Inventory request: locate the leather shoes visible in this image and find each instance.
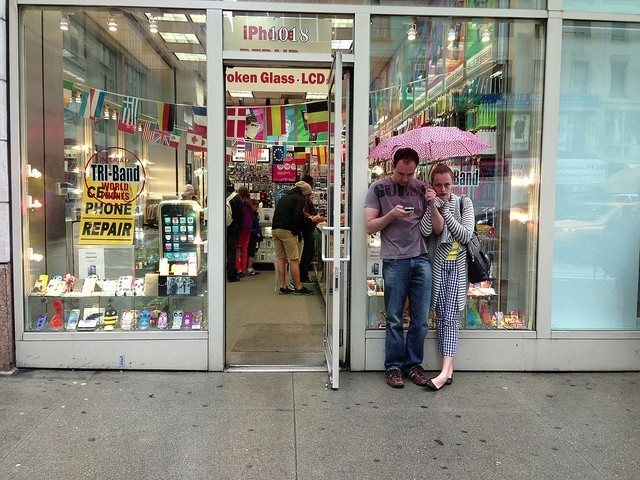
[426,375,444,390]
[445,373,453,385]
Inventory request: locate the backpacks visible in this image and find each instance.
[225,191,238,226]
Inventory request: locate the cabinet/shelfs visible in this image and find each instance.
[312,222,326,306]
[368,285,499,330]
[29,292,206,330]
[254,224,319,270]
[75,229,159,279]
[26,176,43,263]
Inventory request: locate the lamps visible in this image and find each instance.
[148,16,158,33]
[479,23,491,42]
[407,23,417,41]
[102,105,109,120]
[111,110,117,120]
[107,12,118,32]
[66,92,74,102]
[138,122,142,132]
[58,11,69,30]
[447,24,456,41]
[76,90,82,103]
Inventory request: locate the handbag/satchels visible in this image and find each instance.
[466,233,491,284]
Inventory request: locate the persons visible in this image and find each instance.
[299,175,323,285]
[235,187,256,277]
[271,181,324,296]
[226,179,244,282]
[419,163,475,391]
[364,148,433,387]
[246,199,261,276]
[182,184,201,207]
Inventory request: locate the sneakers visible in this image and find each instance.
[288,283,291,289]
[229,276,240,282]
[240,272,245,277]
[291,285,295,290]
[246,268,257,276]
[279,288,294,295]
[293,286,315,295]
[387,370,405,387]
[301,279,317,285]
[405,366,428,385]
[253,268,260,274]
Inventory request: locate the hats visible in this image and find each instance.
[296,180,312,195]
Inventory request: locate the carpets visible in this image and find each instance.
[231,322,326,352]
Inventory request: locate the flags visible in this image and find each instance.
[294,147,306,165]
[118,112,135,134]
[330,102,334,133]
[63,80,73,108]
[186,129,203,152]
[225,138,232,163]
[330,147,334,164]
[153,123,171,147]
[316,146,328,164]
[79,91,96,120]
[272,146,284,164]
[226,108,246,137]
[192,106,207,139]
[265,106,286,136]
[157,102,175,131]
[306,102,328,133]
[83,88,105,119]
[142,121,156,140]
[169,129,182,148]
[201,138,207,152]
[245,142,258,164]
[121,96,140,127]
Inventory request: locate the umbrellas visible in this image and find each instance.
[365,127,493,189]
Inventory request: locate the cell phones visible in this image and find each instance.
[157,199,201,273]
[92,265,96,275]
[87,265,92,276]
[68,309,80,327]
[405,206,415,211]
[374,262,379,275]
